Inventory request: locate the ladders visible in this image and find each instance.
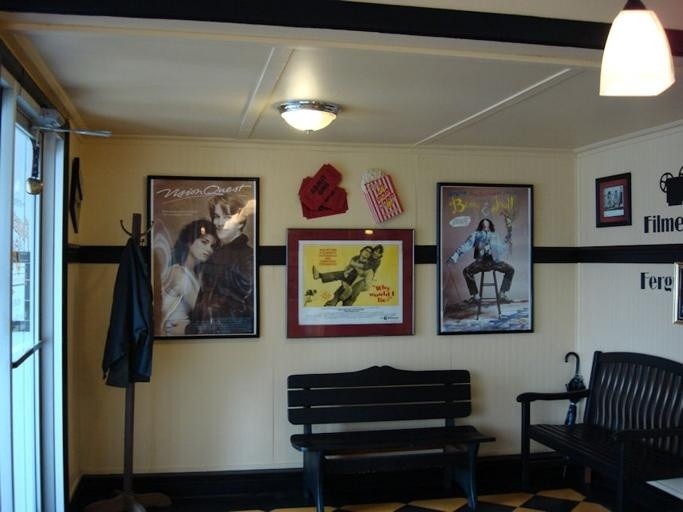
[477,270,501,320]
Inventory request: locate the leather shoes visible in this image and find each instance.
[463,297,480,305]
[497,294,512,303]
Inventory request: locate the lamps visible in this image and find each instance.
[598,0,677,99]
[277,100,339,136]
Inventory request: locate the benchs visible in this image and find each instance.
[516,351,683,512]
[287,365,497,511]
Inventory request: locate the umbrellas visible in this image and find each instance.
[558,352,585,477]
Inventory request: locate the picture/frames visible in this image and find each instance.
[436,182,534,334]
[287,228,415,338]
[595,173,631,227]
[147,175,259,338]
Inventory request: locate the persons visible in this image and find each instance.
[322,246,374,307]
[445,219,519,307]
[310,243,385,300]
[159,218,218,335]
[163,196,253,334]
